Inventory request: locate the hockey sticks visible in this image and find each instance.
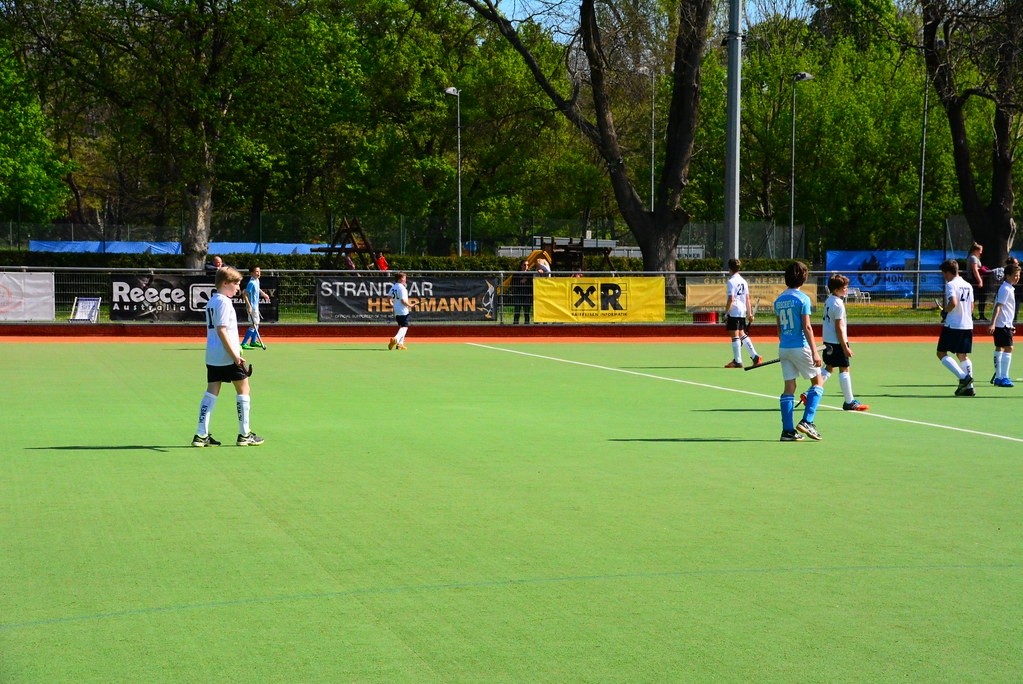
[741,296,761,346]
[241,361,253,377]
[744,344,834,372]
[934,298,943,310]
[250,313,267,350]
[990,327,1016,383]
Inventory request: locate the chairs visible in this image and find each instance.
[825,286,871,304]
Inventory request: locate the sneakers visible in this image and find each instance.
[843,400,869,411]
[250,342,262,347]
[724,359,742,368]
[800,393,807,406]
[388,337,396,350]
[780,429,804,441]
[999,378,1013,387]
[752,355,762,367]
[236,432,265,446]
[396,344,407,349]
[796,419,822,440]
[240,344,254,349]
[994,378,1001,385]
[964,388,976,396]
[192,434,222,446]
[954,375,974,395]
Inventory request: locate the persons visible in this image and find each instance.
[967,242,990,322]
[368,252,391,270]
[1005,256,1018,322]
[987,265,1021,387]
[937,259,975,396]
[241,265,270,350]
[388,272,415,350]
[774,260,825,440]
[191,266,264,447]
[205,256,227,275]
[510,257,584,325]
[346,253,355,269]
[723,259,763,368]
[800,272,869,412]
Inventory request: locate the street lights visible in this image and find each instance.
[789,72,813,258]
[445,87,461,259]
